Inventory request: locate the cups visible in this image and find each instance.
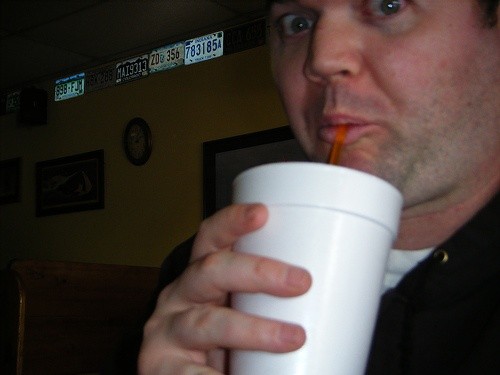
[229,161,404,375]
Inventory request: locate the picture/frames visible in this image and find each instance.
[203,123,312,219]
[0,157,22,205]
[35,149,105,216]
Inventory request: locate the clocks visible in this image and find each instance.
[124,117,153,166]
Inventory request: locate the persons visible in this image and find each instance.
[141,0,499,374]
